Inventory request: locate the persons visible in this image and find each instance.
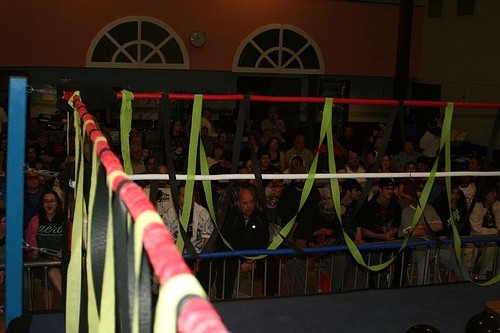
[0,207,7,285]
[0,105,500,224]
[25,190,72,310]
[288,184,348,294]
[356,178,411,287]
[334,178,389,289]
[439,186,473,282]
[162,184,217,295]
[401,187,469,285]
[469,182,500,282]
[215,188,279,299]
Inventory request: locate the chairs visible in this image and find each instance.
[182,238,491,287]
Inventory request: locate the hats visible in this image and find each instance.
[379,179,394,188]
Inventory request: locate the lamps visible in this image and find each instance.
[189,29,206,47]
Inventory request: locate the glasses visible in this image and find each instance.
[43,199,57,205]
